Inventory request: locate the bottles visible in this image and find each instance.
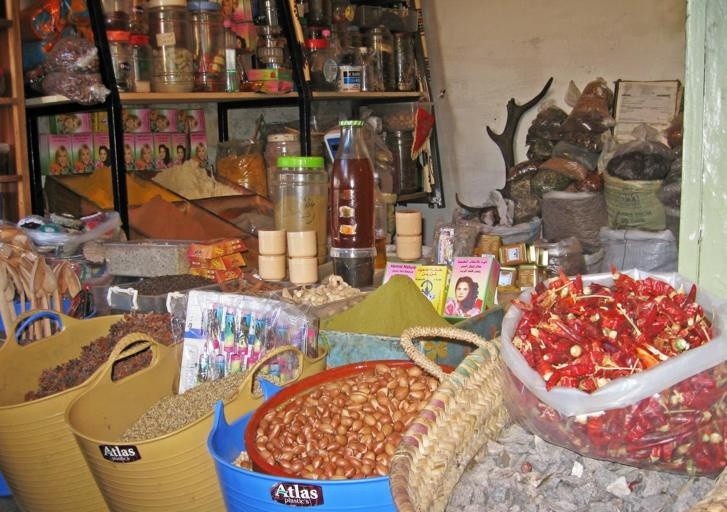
[387,129,419,195]
[304,0,417,92]
[105,1,226,93]
[330,120,375,249]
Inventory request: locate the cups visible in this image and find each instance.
[258,227,318,286]
[395,236,422,261]
[395,209,422,236]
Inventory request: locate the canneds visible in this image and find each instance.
[147,0,239,92]
[335,64,362,92]
[478,235,549,289]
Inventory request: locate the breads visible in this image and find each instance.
[0,223,81,301]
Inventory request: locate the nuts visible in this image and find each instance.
[240,363,441,480]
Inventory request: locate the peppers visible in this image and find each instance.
[507,263,727,477]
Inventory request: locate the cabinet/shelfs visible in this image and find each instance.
[1,0,445,244]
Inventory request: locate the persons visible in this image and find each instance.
[444,277,481,318]
[50,111,206,176]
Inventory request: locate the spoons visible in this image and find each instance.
[0,257,83,342]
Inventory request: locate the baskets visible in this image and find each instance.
[390,326,727,512]
